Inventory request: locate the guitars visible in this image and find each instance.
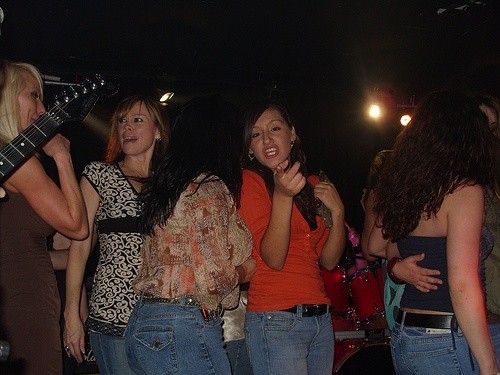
[0,74,120,199]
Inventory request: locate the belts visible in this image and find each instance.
[142,293,198,306]
[277,303,333,319]
[396,308,460,333]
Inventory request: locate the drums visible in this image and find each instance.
[332,338,396,375]
[319,265,388,328]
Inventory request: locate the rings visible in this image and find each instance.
[64,345,70,353]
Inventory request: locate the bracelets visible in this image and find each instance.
[386,257,406,285]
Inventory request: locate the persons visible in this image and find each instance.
[232,102,345,375]
[62,95,171,375]
[48,232,99,324]
[124,102,258,375]
[367,93,500,375]
[344,147,407,336]
[385,96,500,371]
[0,62,89,375]
